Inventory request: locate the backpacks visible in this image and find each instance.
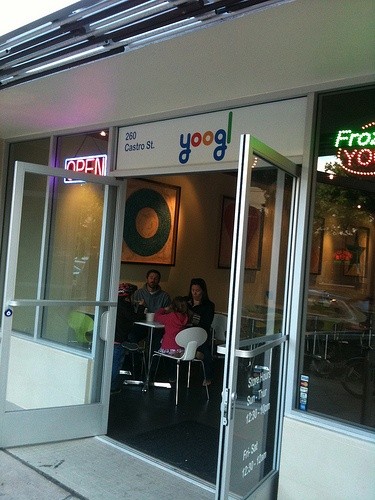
[118,283,138,302]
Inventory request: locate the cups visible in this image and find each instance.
[147,313,155,321]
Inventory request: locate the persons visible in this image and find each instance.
[104,270,217,395]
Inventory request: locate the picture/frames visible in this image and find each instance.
[343,225,370,278]
[218,195,264,271]
[310,218,325,275]
[116,177,181,266]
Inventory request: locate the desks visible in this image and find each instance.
[76,304,375,392]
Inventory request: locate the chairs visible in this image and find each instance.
[141,327,209,406]
[66,311,94,350]
[210,314,227,359]
